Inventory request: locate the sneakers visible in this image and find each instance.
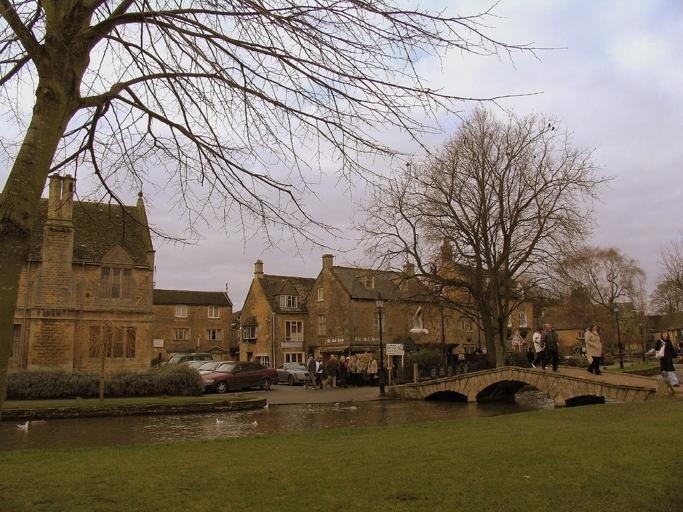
[531,362,536,368]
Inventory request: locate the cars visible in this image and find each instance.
[645,348,656,355]
[198,360,280,394]
[272,361,310,386]
[167,352,226,378]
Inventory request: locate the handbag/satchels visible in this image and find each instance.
[656,345,665,360]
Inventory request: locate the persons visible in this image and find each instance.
[585,323,603,374]
[530,327,549,369]
[303,351,398,390]
[541,321,562,373]
[593,321,601,375]
[655,330,681,387]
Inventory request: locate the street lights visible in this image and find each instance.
[372,291,388,396]
[433,282,448,370]
[613,302,626,369]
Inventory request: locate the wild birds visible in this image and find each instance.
[215,417,224,424]
[252,420,258,426]
[263,403,269,409]
[15,420,31,432]
